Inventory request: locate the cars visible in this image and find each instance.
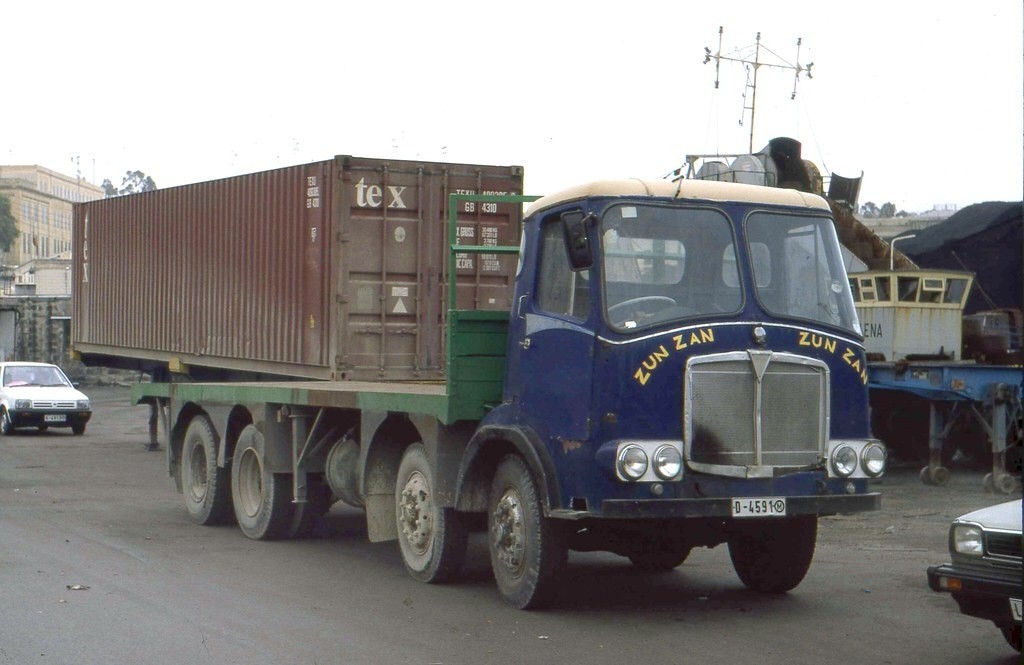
[927,497,1024,651]
[0,362,93,434]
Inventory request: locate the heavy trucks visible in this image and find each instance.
[67,154,886,613]
